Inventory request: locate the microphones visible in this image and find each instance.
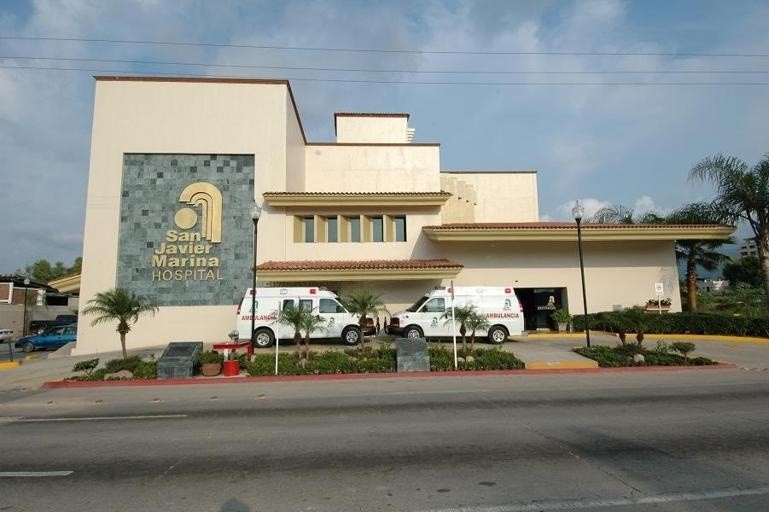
[223,360,240,376]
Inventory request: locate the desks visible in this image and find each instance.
[246,199,262,355]
[570,200,591,347]
[23,278,30,337]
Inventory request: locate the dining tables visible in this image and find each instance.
[197,350,222,376]
[551,309,572,331]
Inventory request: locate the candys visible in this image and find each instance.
[383,285,524,345]
[233,286,381,346]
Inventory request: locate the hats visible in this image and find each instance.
[15,325,79,354]
[0,327,14,344]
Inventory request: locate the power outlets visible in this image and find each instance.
[30,315,78,335]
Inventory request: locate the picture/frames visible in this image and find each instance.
[212,340,253,361]
